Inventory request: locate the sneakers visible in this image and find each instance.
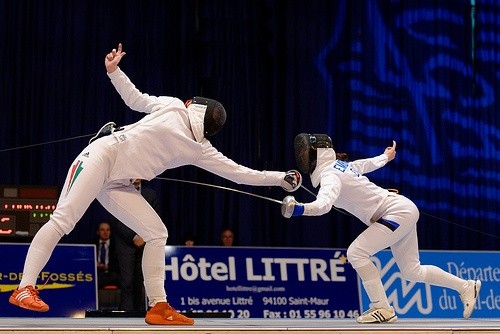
[144,302,195,325]
[458,280,481,319]
[8,285,50,313]
[356,307,398,324]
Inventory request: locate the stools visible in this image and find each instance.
[99,284,121,311]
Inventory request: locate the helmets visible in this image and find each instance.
[295,133,333,173]
[192,96,226,138]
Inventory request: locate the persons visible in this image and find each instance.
[93,180,160,311]
[183,235,197,245]
[221,229,234,246]
[292,134,481,323]
[9,44,297,325]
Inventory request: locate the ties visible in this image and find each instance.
[100,243,106,268]
